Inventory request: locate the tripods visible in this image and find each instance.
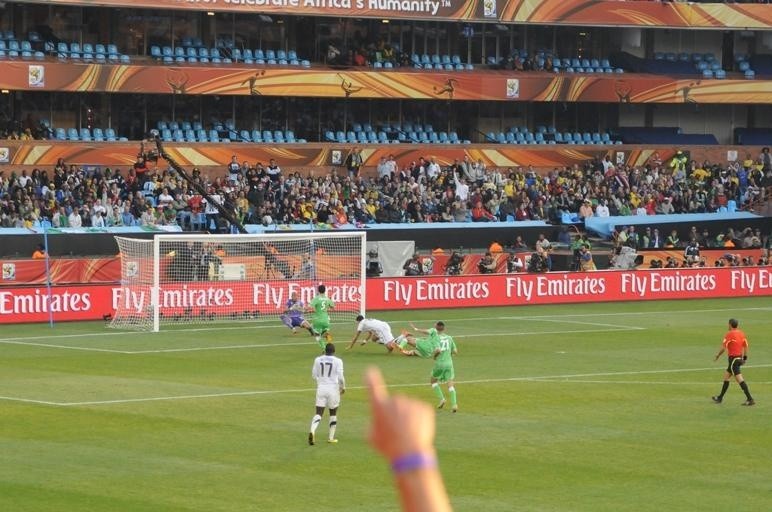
[258,262,278,281]
[570,255,584,271]
[574,259,585,272]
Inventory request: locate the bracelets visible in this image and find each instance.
[391,447,443,474]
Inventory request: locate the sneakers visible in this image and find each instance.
[740,400,755,406]
[451,406,457,413]
[712,396,720,403]
[437,400,446,409]
[328,439,338,443]
[308,433,314,446]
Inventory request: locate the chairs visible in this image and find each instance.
[1,113,772,146]
[1,34,772,82]
[0,168,772,271]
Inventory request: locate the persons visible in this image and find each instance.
[513,53,552,74]
[308,341,346,446]
[571,235,584,271]
[507,252,523,272]
[165,242,226,281]
[477,253,497,274]
[134,154,225,232]
[512,235,528,249]
[30,243,50,259]
[685,241,700,258]
[0,156,135,228]
[448,251,459,264]
[261,242,281,259]
[351,32,397,71]
[367,252,384,277]
[663,229,681,248]
[633,254,645,265]
[224,147,363,232]
[279,291,313,336]
[699,226,712,248]
[289,253,315,278]
[577,244,597,271]
[714,249,772,268]
[536,234,554,271]
[488,240,504,254]
[316,243,329,254]
[363,154,544,223]
[687,226,701,243]
[3,112,57,140]
[363,363,451,512]
[394,323,448,359]
[641,226,652,249]
[582,234,591,251]
[714,224,772,250]
[616,226,629,255]
[431,245,444,255]
[115,247,129,259]
[544,147,772,225]
[649,228,663,249]
[303,285,335,355]
[404,253,424,276]
[327,41,340,60]
[430,322,457,413]
[649,256,707,269]
[626,226,639,249]
[346,315,422,358]
[712,318,755,406]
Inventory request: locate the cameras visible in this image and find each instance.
[572,248,584,257]
[650,258,664,267]
[668,258,678,266]
[714,260,720,266]
[369,262,379,275]
[451,253,464,275]
[478,261,487,272]
[727,254,733,263]
[528,251,547,273]
[611,244,645,269]
[134,142,157,168]
[505,255,516,274]
[742,258,748,263]
[759,258,764,264]
[699,260,707,266]
[682,258,689,266]
